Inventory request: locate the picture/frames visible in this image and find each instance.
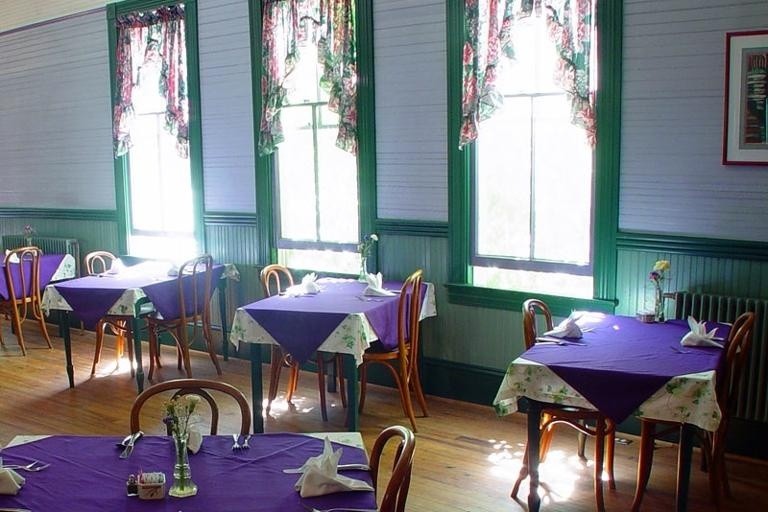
[720,27,767,167]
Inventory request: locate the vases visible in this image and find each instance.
[167,434,200,501]
[356,258,368,284]
[652,292,669,322]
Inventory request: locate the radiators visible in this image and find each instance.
[4,233,82,283]
[665,290,768,424]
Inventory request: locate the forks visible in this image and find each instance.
[1,458,53,471]
[669,345,716,356]
[231,431,252,450]
[297,502,377,512]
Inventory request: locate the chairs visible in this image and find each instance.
[627,306,757,510]
[511,295,623,511]
[141,253,225,381]
[83,249,164,379]
[256,263,350,425]
[368,422,421,510]
[4,246,56,356]
[127,376,255,440]
[335,267,432,433]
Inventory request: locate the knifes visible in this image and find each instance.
[119,431,139,459]
[282,462,376,476]
[533,336,589,347]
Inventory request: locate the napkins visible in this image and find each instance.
[359,270,400,300]
[281,432,375,504]
[3,462,24,501]
[295,268,320,295]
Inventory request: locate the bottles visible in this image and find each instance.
[125,473,139,497]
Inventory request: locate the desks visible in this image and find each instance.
[2,251,78,342]
[228,274,432,434]
[39,258,238,391]
[3,430,376,511]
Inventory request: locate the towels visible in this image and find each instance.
[677,314,725,354]
[541,309,586,345]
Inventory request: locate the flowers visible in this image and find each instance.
[157,391,204,432]
[356,232,378,257]
[647,257,674,293]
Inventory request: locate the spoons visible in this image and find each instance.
[116,434,131,447]
